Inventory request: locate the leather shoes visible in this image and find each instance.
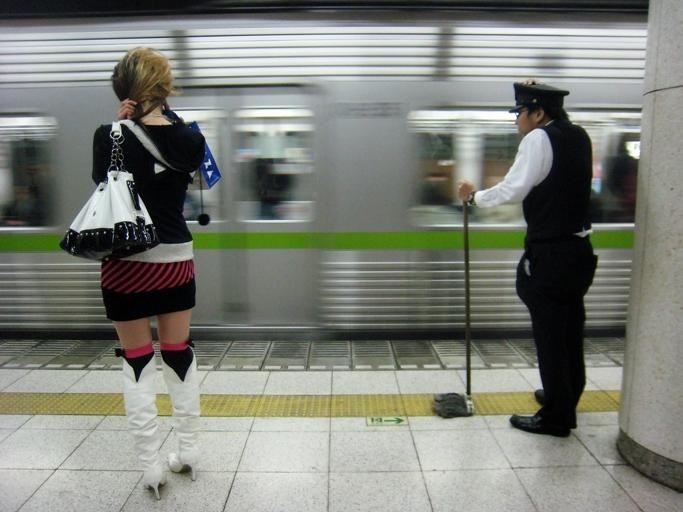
[510,413,569,437]
[535,389,576,428]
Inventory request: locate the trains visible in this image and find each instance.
[0,18,649,330]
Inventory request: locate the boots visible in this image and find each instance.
[159,345,202,481]
[121,347,167,500]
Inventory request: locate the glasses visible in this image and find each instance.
[514,108,534,116]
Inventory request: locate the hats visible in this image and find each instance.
[508,82,569,113]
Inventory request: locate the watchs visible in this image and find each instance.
[468,190,475,205]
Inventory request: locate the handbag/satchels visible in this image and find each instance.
[59,170,160,262]
[187,121,222,190]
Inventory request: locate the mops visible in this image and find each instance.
[432,200,479,418]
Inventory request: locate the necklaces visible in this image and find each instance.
[145,114,166,118]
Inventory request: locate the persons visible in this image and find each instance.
[90,45,205,500]
[457,83,599,437]
